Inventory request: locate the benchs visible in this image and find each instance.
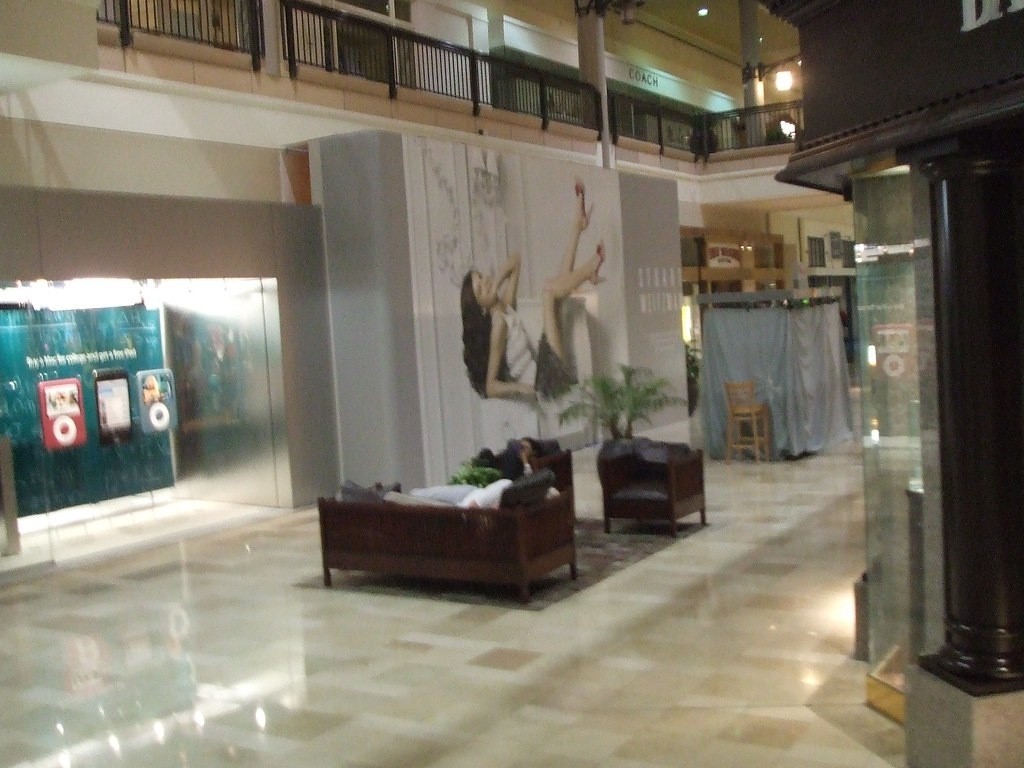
[315,483,580,606]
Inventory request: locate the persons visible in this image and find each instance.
[410,479,560,509]
[461,176,608,399]
[478,437,544,482]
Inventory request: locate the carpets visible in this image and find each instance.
[295,517,708,613]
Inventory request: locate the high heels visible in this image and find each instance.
[588,240,607,286]
[574,183,594,229]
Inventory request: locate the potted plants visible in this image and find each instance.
[558,363,693,485]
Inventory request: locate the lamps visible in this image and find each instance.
[774,61,793,91]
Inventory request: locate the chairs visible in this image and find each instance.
[508,437,573,492]
[723,381,771,464]
[599,440,707,537]
